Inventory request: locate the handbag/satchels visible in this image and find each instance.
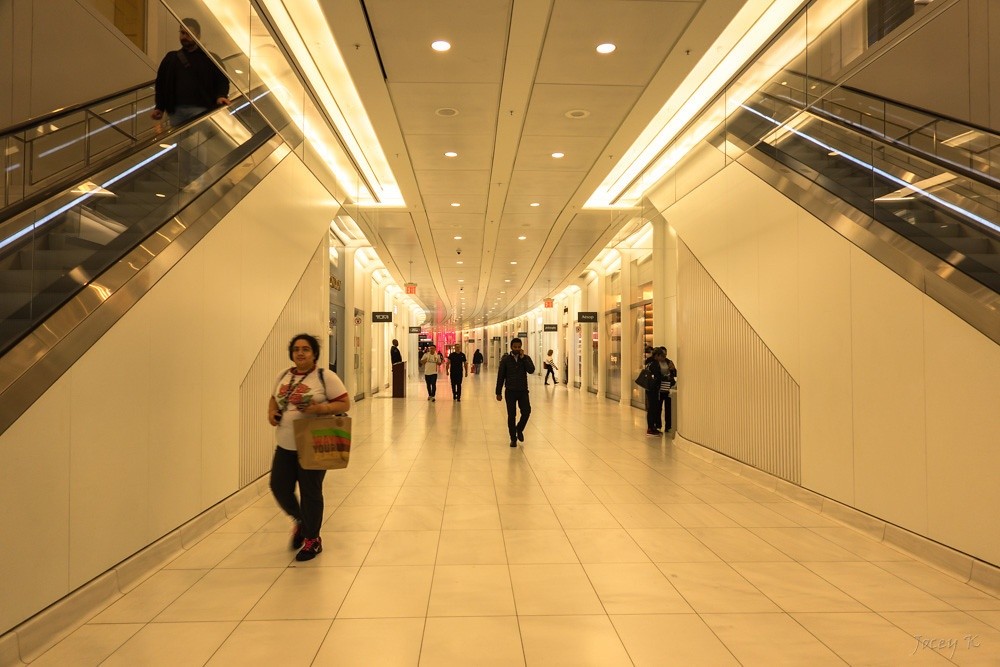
[292,369,352,470]
[544,362,548,369]
[635,364,650,391]
[471,365,476,373]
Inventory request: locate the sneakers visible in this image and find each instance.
[296,537,322,561]
[292,523,305,549]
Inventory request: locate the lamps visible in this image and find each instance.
[544,280,554,308]
[404,261,416,294]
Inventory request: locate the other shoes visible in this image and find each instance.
[428,396,431,400]
[453,394,456,399]
[555,381,559,384]
[647,429,663,436]
[457,396,460,401]
[666,429,674,433]
[545,382,550,385]
[510,441,517,447]
[516,427,524,441]
[431,397,435,402]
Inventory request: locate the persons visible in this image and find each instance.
[419,347,429,367]
[446,345,468,401]
[496,338,535,447]
[421,345,442,402]
[438,350,444,366]
[473,349,483,374]
[391,339,402,364]
[644,346,677,436]
[268,334,350,561]
[152,17,231,128]
[545,350,559,385]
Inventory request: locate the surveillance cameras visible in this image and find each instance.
[456,250,461,254]
